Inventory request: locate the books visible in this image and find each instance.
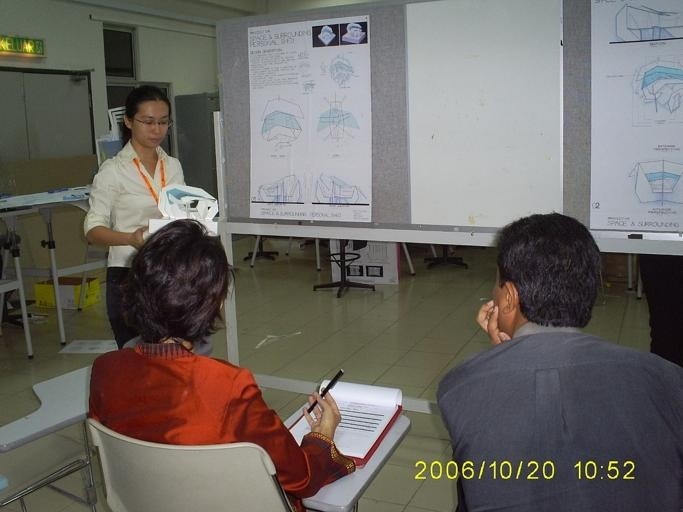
[288,378,402,469]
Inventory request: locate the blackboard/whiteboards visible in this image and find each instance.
[403,1,565,229]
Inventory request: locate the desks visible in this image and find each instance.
[281,396,412,511]
[0,363,95,457]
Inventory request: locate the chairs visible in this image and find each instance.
[84,416,294,512]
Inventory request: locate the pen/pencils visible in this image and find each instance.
[306,368,345,415]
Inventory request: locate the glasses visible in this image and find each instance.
[128,115,175,129]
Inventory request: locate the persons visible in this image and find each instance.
[85,84,187,350]
[90,219,357,511]
[436,211,682,511]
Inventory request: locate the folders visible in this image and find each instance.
[303,397,402,470]
